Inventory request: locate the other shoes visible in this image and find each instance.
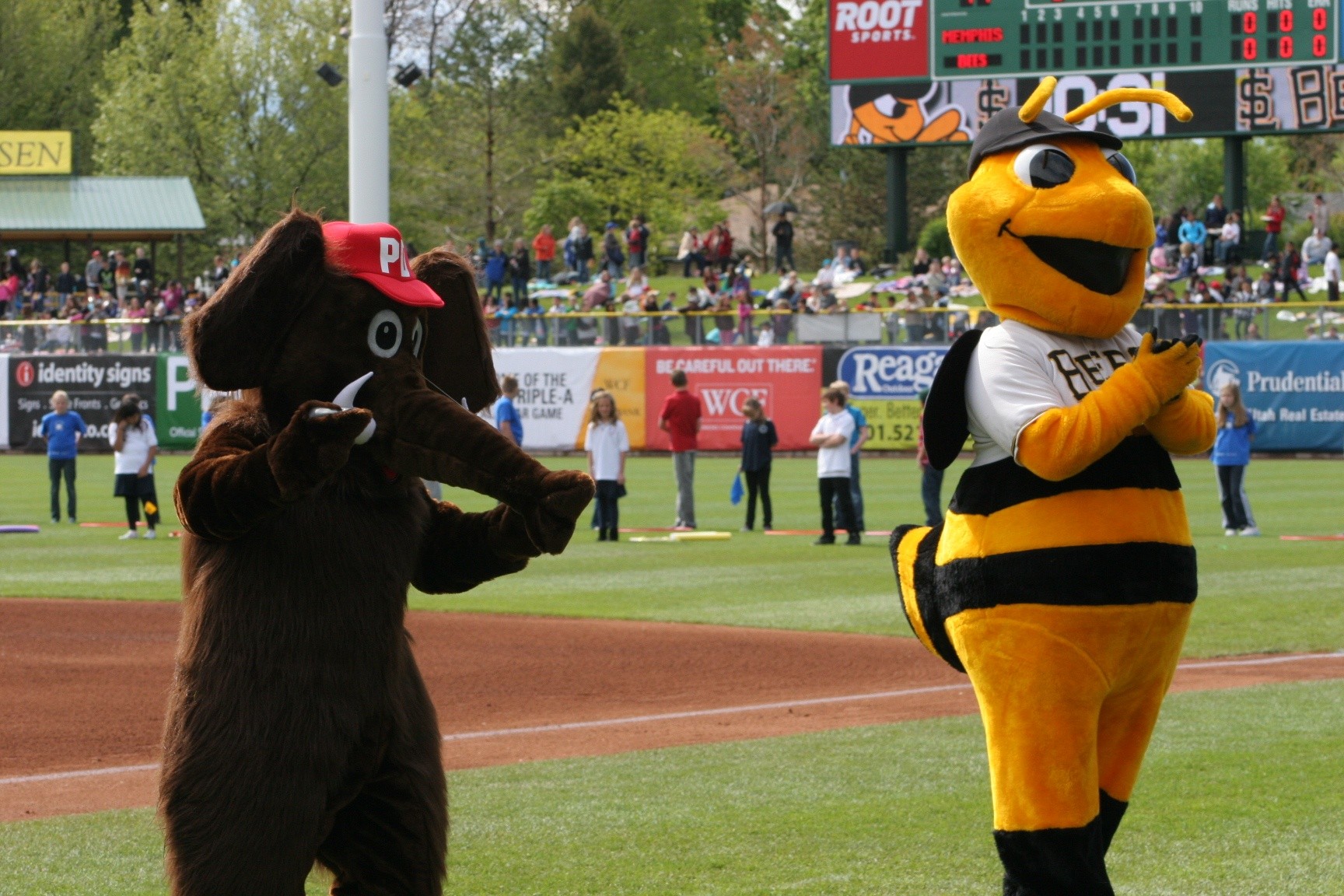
[764,523,771,530]
[50,517,59,523]
[69,517,75,523]
[857,520,865,530]
[815,535,835,545]
[1225,527,1237,536]
[675,525,695,531]
[741,526,753,532]
[1240,526,1260,537]
[845,534,862,544]
[118,529,137,540]
[593,526,600,531]
[142,529,156,539]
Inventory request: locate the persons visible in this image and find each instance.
[108,395,162,541]
[584,387,629,542]
[40,390,86,524]
[808,380,868,545]
[915,390,944,527]
[740,398,779,531]
[494,376,523,448]
[0,193,1344,355]
[658,370,703,529]
[1209,382,1260,537]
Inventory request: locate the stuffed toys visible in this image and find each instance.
[888,74,1216,895]
[158,208,596,896]
